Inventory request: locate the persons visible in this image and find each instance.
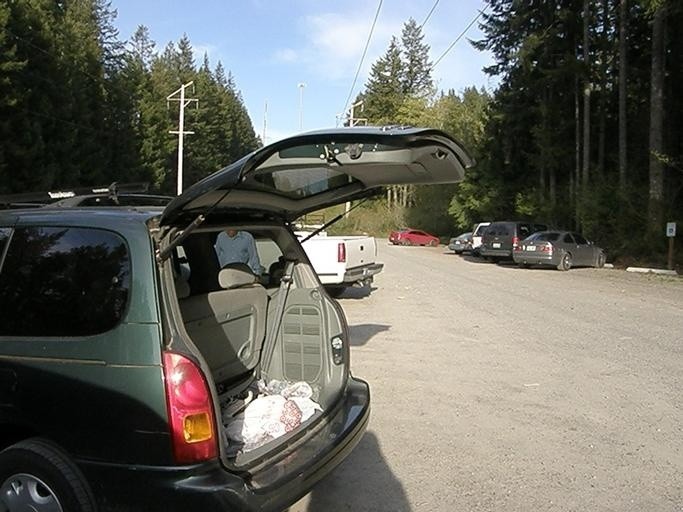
[215,231,266,274]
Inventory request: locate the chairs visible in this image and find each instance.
[173,262,269,386]
